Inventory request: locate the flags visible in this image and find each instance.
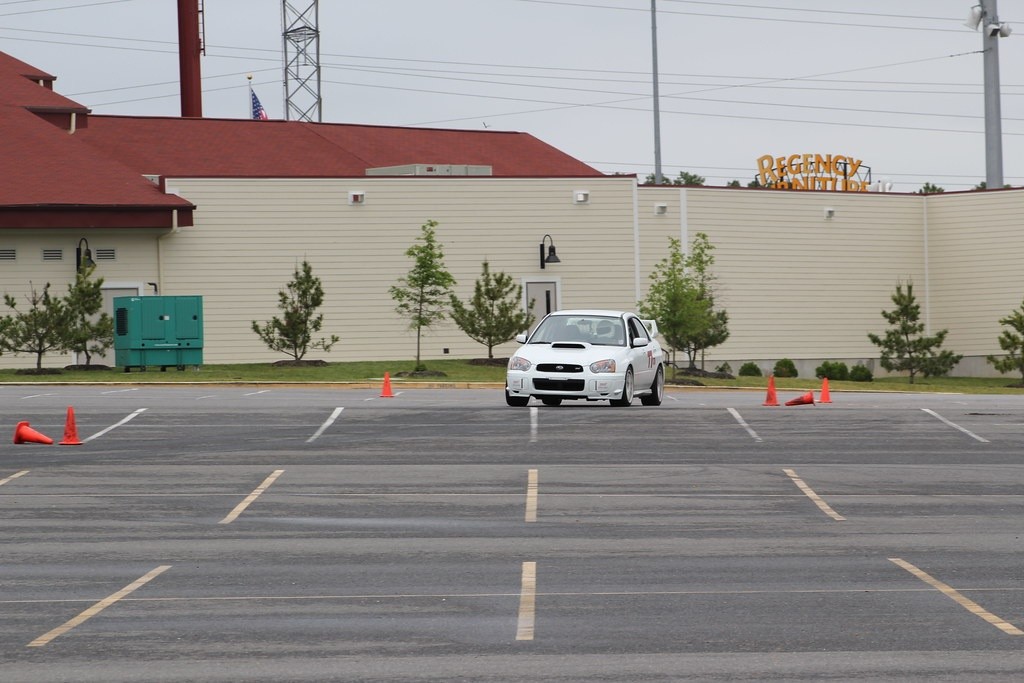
[252,88,268,119]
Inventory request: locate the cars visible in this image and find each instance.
[505,310,666,408]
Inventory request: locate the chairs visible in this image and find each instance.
[614,324,623,340]
[563,324,580,341]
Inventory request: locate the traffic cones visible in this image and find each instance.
[814,378,832,404]
[784,389,816,406]
[761,375,781,406]
[378,372,396,397]
[57,404,85,445]
[12,420,54,445]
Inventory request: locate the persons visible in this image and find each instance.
[594,320,617,343]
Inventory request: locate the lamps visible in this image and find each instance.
[76,237,96,274]
[540,234,560,269]
